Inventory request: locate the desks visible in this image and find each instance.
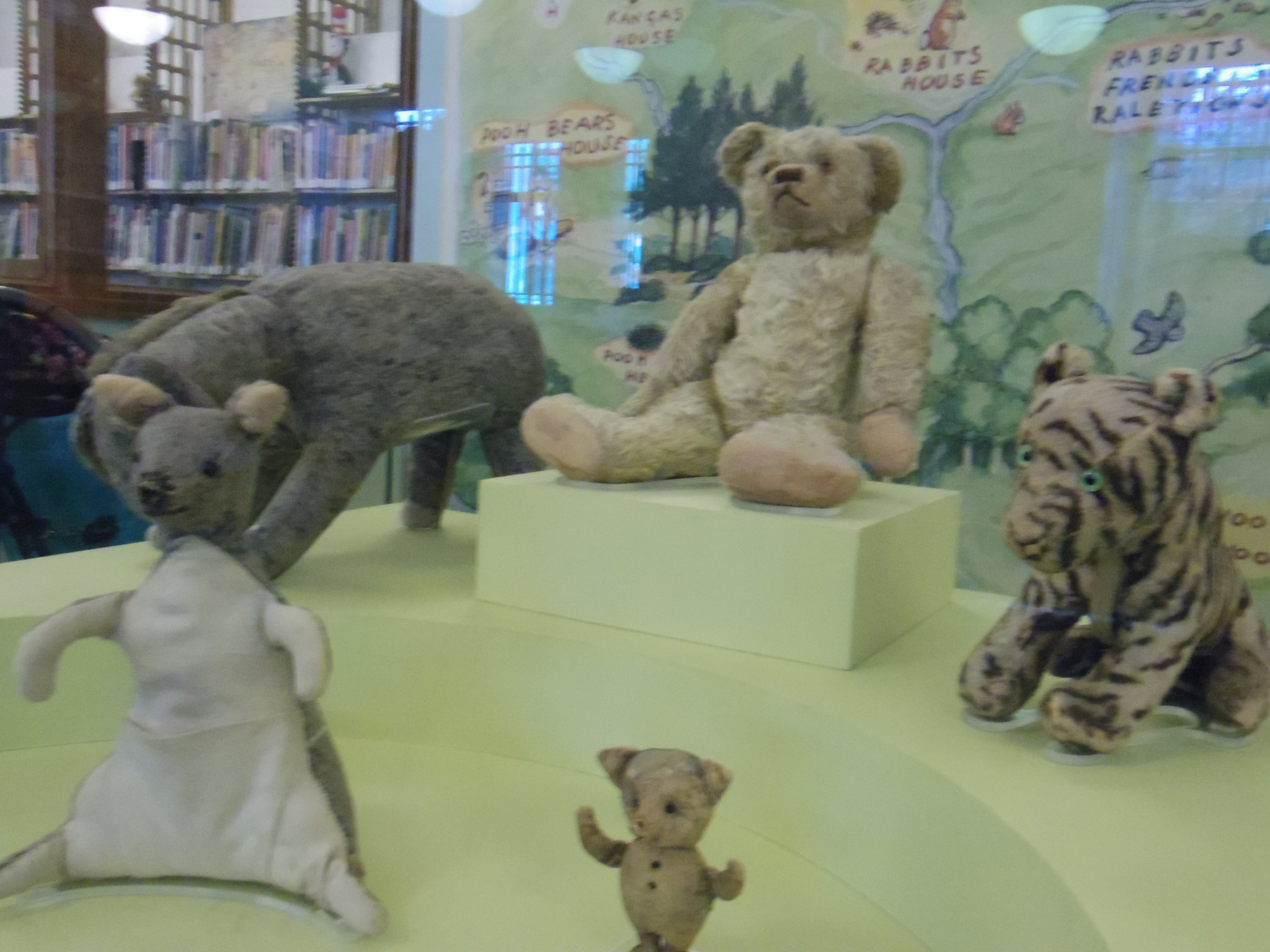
[0,497,1269,952]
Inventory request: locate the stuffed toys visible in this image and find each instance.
[518,123,933,509]
[0,374,386,934]
[578,747,743,951]
[78,257,552,580]
[957,338,1266,758]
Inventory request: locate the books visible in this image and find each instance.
[0,114,398,297]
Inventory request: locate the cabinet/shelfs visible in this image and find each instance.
[0,186,40,276]
[99,180,396,292]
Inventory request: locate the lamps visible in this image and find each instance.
[1018,2,1107,56]
[572,46,645,86]
[92,3,172,50]
[418,1,480,18]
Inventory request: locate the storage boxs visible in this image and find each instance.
[469,464,959,672]
